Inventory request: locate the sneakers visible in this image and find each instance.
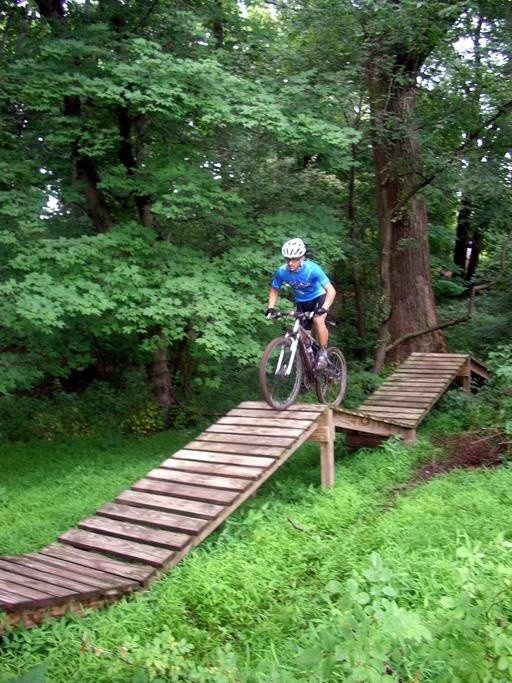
[315,350,329,371]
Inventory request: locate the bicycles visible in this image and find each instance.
[259,310,348,411]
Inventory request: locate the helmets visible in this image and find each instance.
[281,238,307,260]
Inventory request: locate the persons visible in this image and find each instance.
[265,238,337,394]
